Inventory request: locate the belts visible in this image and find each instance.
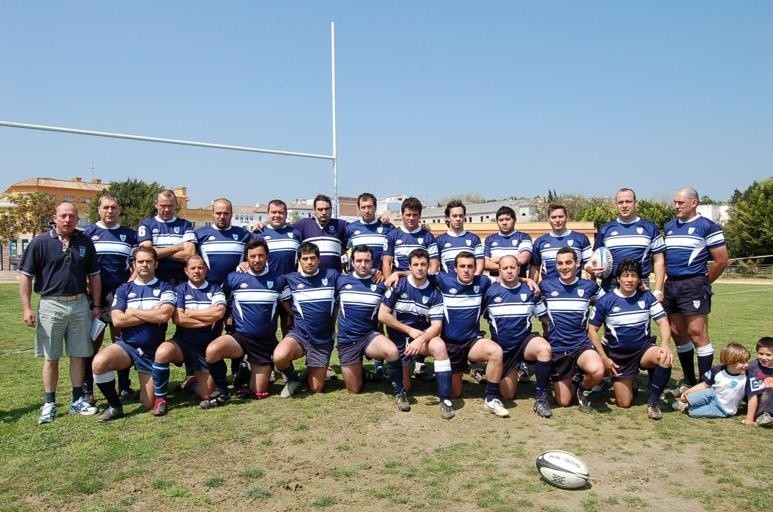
[50,295,84,302]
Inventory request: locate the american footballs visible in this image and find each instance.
[536,449,590,489]
[591,247,613,279]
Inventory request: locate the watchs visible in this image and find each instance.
[91,303,99,307]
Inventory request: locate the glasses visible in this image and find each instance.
[64,246,72,265]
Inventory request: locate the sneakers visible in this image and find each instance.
[644,405,665,421]
[587,376,612,390]
[96,407,125,420]
[533,401,552,419]
[373,371,384,381]
[483,398,511,420]
[39,403,56,424]
[281,380,300,395]
[323,366,337,382]
[112,390,137,399]
[756,413,772,426]
[516,368,529,383]
[150,396,168,416]
[576,389,593,412]
[408,371,432,384]
[199,392,232,409]
[467,368,487,384]
[70,397,97,415]
[663,384,692,400]
[82,386,94,402]
[394,391,409,411]
[229,362,250,389]
[437,399,456,418]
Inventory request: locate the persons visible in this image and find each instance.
[91,244,178,423]
[344,192,431,376]
[530,246,608,415]
[79,191,138,405]
[248,199,305,382]
[664,186,732,397]
[746,336,773,427]
[380,197,438,384]
[198,237,292,410]
[482,205,533,385]
[585,187,666,397]
[251,194,392,381]
[530,203,597,397]
[377,248,456,420]
[332,244,411,412]
[587,258,675,419]
[137,189,197,396]
[381,251,540,419]
[434,198,485,386]
[485,257,552,419]
[671,342,763,427]
[15,201,103,425]
[194,197,254,395]
[235,240,342,398]
[148,254,226,417]
